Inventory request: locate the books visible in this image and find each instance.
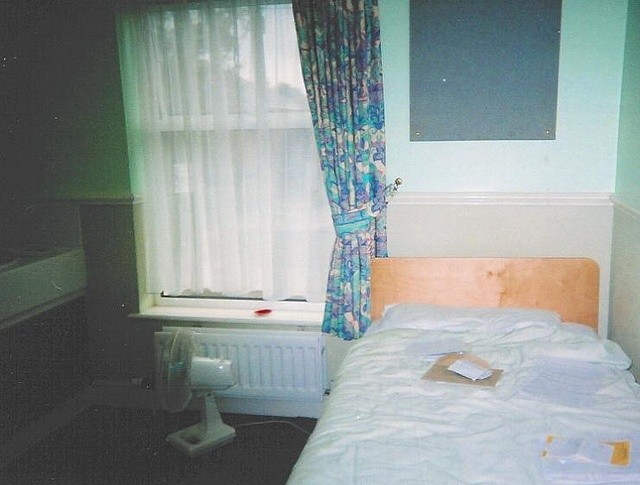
[420,360,505,388]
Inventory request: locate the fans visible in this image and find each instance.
[153,331,238,457]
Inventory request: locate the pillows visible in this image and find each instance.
[381,302,559,338]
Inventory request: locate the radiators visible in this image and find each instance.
[161,325,325,405]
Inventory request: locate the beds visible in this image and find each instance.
[290,258,638,483]
